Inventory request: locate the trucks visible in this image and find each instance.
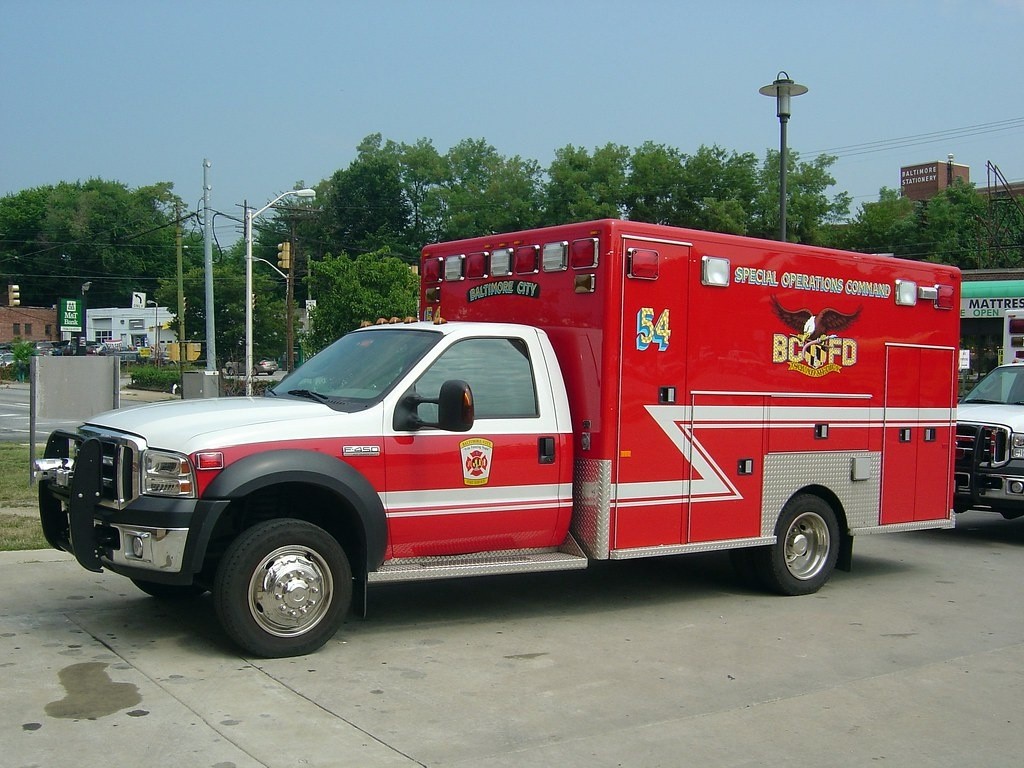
[954,307,1024,518]
[32,217,961,660]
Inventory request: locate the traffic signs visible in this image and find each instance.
[132,292,146,309]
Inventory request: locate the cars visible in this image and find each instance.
[0,338,170,369]
[225,353,279,376]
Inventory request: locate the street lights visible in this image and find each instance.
[146,300,159,368]
[244,188,316,397]
[240,253,295,374]
[759,70,808,244]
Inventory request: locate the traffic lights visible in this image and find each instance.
[182,297,189,315]
[8,285,21,307]
[278,241,290,268]
